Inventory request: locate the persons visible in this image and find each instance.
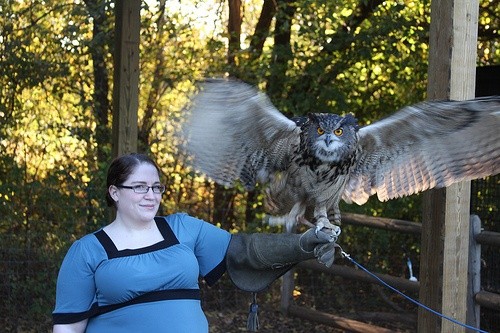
[53,153,335,333]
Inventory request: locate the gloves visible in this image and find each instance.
[226,228,335,291]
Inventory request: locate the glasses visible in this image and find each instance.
[116,185,165,194]
